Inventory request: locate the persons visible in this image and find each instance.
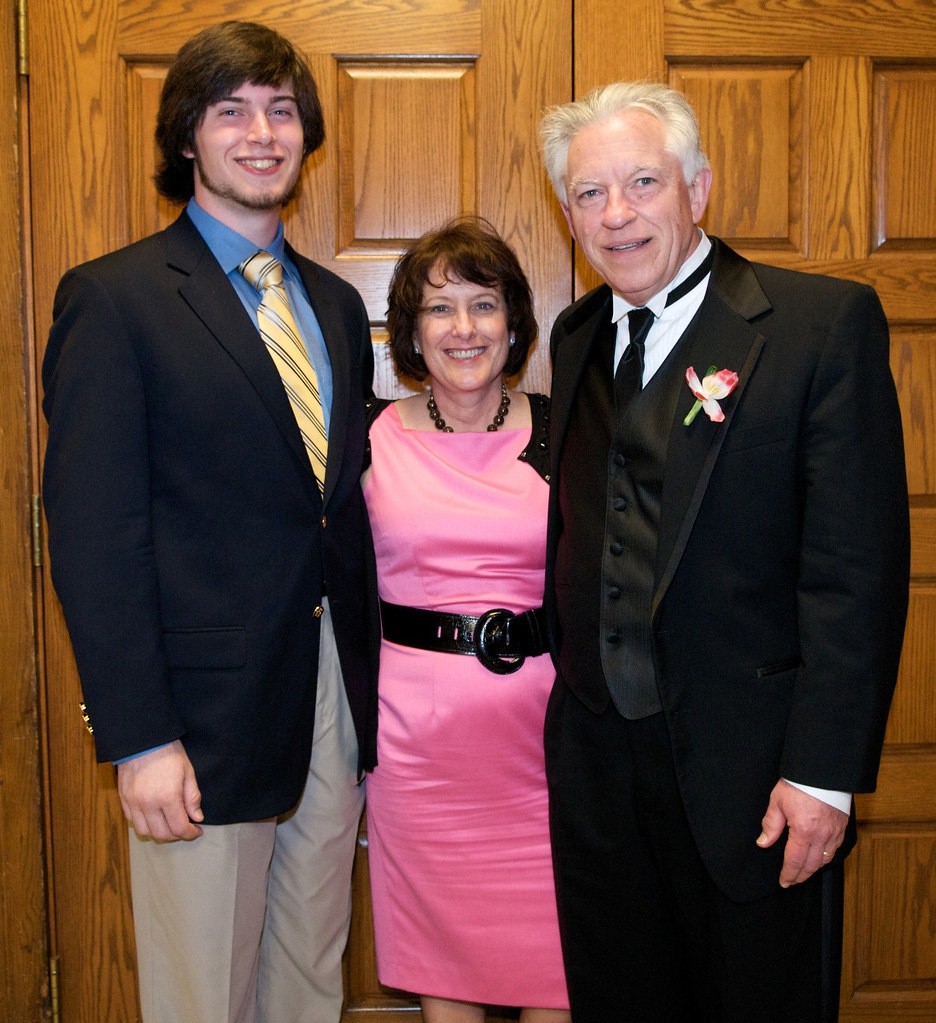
[41,19,381,1023]
[362,216,571,1023]
[539,82,910,1023]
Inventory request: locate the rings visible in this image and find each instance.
[823,851,833,857]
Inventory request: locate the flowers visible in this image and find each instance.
[683,365,739,426]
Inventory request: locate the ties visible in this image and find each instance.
[614,244,714,421]
[238,250,327,499]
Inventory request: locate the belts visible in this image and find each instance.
[379,596,550,675]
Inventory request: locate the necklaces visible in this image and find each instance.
[427,384,511,433]
